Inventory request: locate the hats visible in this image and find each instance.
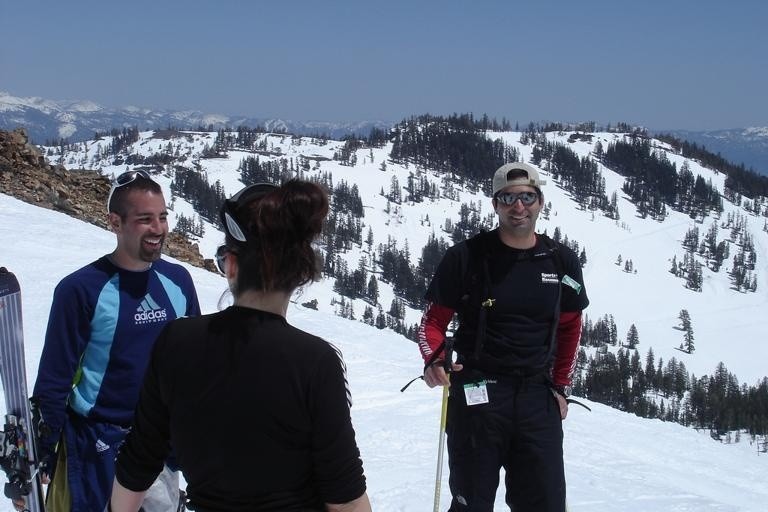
[491,162,549,197]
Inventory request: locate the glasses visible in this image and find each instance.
[214,244,241,276]
[108,169,152,214]
[496,191,540,208]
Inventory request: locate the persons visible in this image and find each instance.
[109,178,372,512]
[415,161,590,511]
[7,168,201,511]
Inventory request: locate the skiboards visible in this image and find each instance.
[0,266,45,511]
